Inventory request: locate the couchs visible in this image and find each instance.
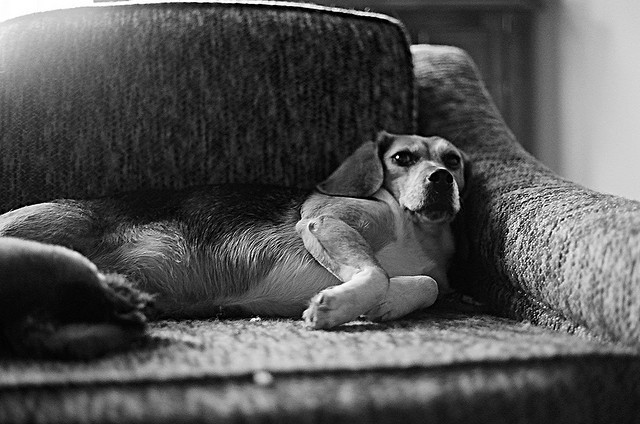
[0,1,636,422]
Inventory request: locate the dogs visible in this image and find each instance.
[0,129,472,330]
